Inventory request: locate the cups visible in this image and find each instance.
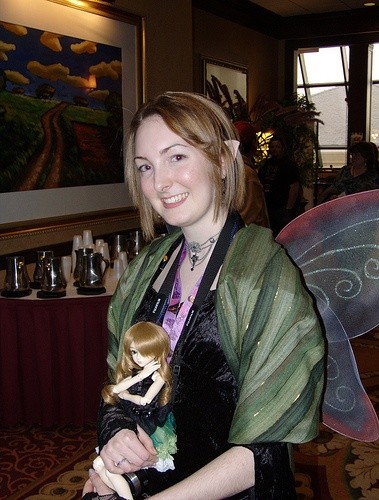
[83,227,93,249]
[95,239,105,254]
[72,235,82,271]
[100,242,109,260]
[120,252,128,268]
[60,257,71,281]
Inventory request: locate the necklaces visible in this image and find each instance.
[185,231,221,271]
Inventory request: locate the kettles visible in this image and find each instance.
[77,253,109,295]
[31,251,56,290]
[74,247,94,286]
[37,258,68,299]
[0,255,32,296]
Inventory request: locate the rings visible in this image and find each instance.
[115,458,126,467]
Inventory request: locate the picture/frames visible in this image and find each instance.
[0,0,149,235]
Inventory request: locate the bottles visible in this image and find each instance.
[113,235,124,280]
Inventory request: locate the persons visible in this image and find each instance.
[228,119,379,230]
[93,321,175,499]
[82,92,327,499]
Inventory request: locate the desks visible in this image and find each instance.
[0,269,129,429]
